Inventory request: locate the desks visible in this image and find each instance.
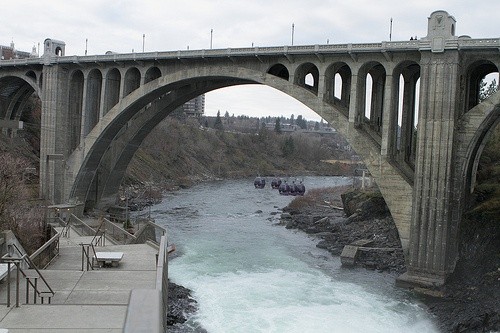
[95,251,124,267]
